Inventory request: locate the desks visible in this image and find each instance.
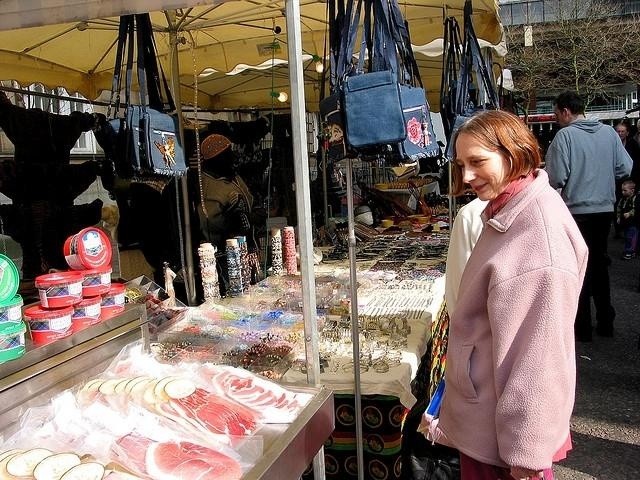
[167,208,449,478]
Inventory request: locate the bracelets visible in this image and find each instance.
[342,314,411,374]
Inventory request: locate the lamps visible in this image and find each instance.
[313,55,324,73]
[272,91,288,101]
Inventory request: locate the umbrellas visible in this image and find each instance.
[1,1,507,307]
[124,23,509,229]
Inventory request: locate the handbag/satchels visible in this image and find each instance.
[382,84,439,161]
[319,93,355,161]
[132,105,187,178]
[343,68,407,146]
[107,116,127,178]
[444,114,471,164]
[124,105,147,175]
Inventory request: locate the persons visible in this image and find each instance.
[195,133,269,296]
[542,87,635,345]
[429,109,591,480]
[614,179,640,262]
[612,118,640,242]
[442,194,492,324]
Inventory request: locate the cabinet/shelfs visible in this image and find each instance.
[0,302,338,479]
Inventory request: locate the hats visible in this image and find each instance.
[201,134,230,159]
